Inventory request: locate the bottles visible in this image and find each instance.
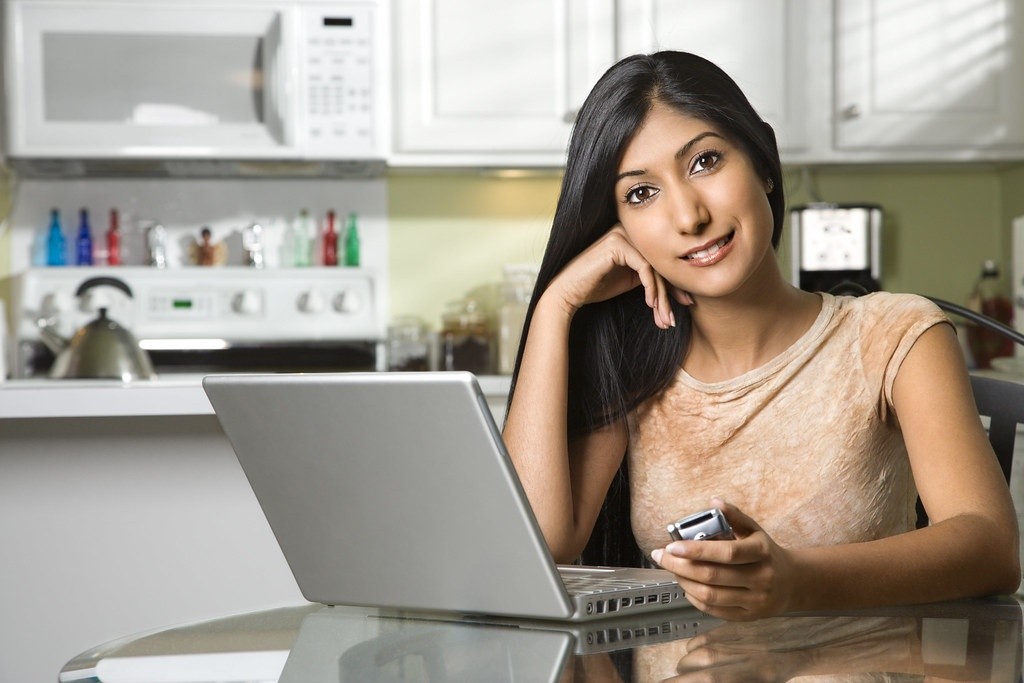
[75,209,94,265]
[294,211,314,266]
[323,211,341,266]
[107,208,122,266]
[965,260,1015,371]
[344,214,361,265]
[46,208,66,265]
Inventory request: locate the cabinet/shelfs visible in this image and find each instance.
[377,0,1024,170]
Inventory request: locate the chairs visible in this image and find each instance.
[915,370,1024,596]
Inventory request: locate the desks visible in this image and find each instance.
[58,603,1024,683]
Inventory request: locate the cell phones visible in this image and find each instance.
[667,506,737,541]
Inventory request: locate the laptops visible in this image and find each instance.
[202,370,695,622]
[278,605,724,683]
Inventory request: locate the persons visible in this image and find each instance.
[501,48,1022,623]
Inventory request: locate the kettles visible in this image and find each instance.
[37,276,154,381]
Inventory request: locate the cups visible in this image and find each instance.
[440,302,491,375]
[387,323,433,374]
